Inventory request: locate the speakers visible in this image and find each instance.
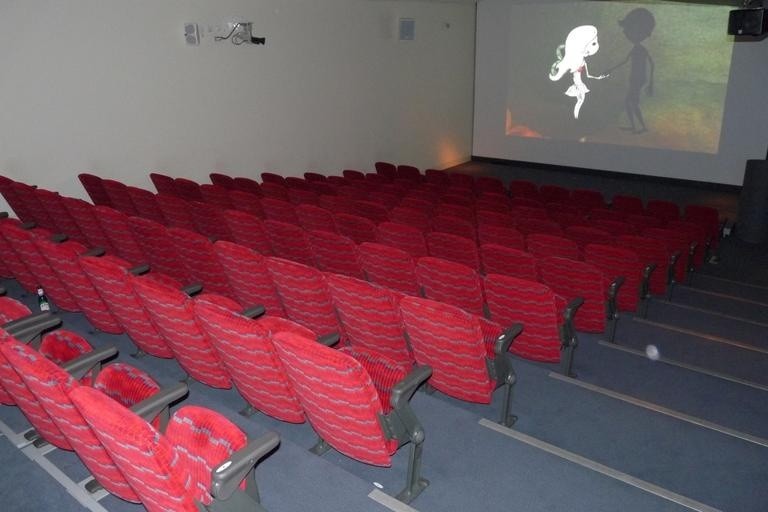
[727,6,768,35]
[184,23,201,48]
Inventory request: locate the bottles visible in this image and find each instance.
[36,285,52,314]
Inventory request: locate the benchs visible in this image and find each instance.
[1,162,725,512]
